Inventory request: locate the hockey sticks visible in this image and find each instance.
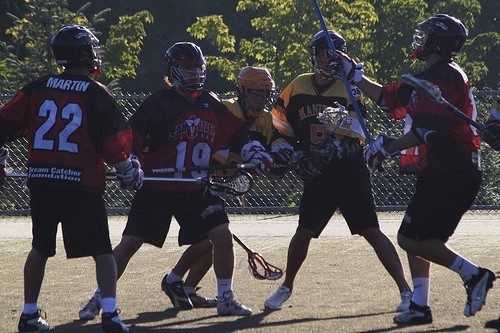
[105,148,326,184]
[315,105,350,141]
[312,0,385,173]
[400,73,500,141]
[5,172,255,197]
[232,232,284,282]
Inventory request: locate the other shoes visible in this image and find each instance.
[396,293,413,311]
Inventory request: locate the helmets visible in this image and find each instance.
[235,66,279,93]
[166,42,207,92]
[410,13,469,62]
[51,24,102,76]
[308,28,348,80]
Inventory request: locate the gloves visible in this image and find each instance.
[481,121,500,151]
[114,159,145,191]
[290,150,323,182]
[324,48,364,85]
[270,136,295,164]
[362,133,402,172]
[0,147,9,186]
[310,139,346,166]
[240,140,276,177]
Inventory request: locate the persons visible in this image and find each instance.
[160,66,297,311]
[262,28,413,311]
[78,42,252,320]
[0,25,144,333]
[484,93,500,150]
[327,14,497,325]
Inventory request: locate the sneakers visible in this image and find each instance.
[160,274,194,310]
[188,287,217,308]
[464,267,497,317]
[78,291,102,320]
[393,299,433,325]
[101,308,130,333]
[17,309,50,333]
[263,285,293,310]
[216,289,252,316]
